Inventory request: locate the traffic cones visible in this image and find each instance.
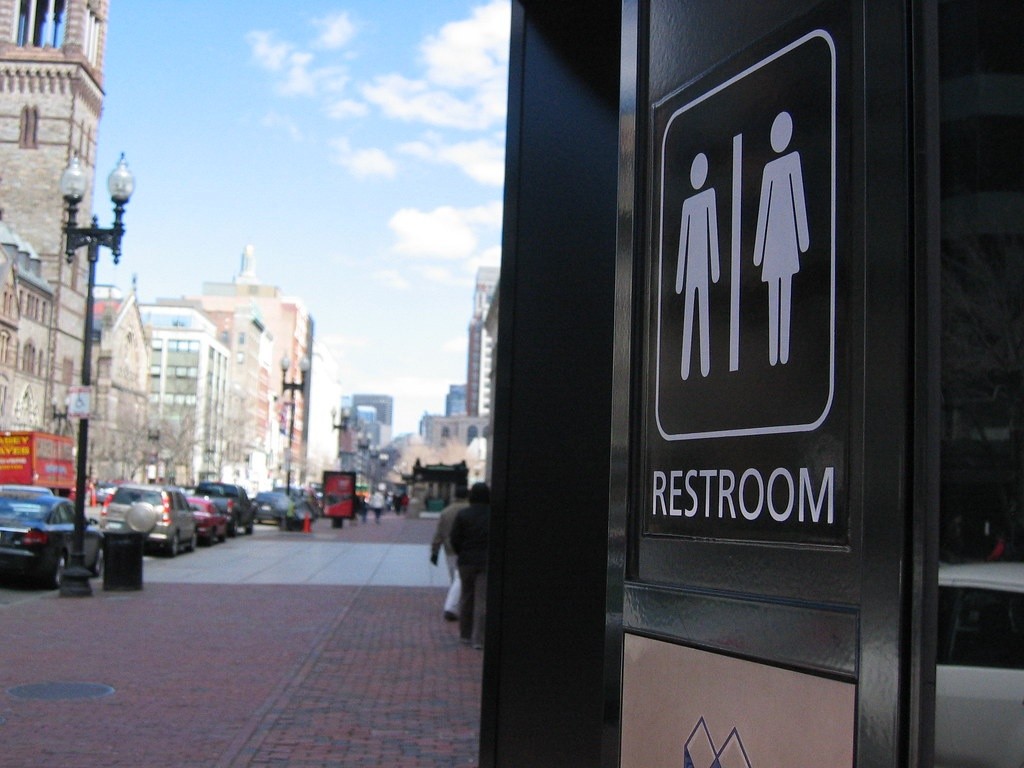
[301,510,313,533]
[90,488,97,507]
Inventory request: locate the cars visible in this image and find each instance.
[0,493,104,589]
[0,484,54,495]
[252,491,292,522]
[187,496,230,546]
[97,483,116,504]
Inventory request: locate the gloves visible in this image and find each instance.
[431,554,437,564]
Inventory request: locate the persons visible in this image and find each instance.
[430,481,489,649]
[357,487,409,525]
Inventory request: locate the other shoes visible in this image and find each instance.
[444,611,458,621]
[459,637,471,644]
[472,642,482,649]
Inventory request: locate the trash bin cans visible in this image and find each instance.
[102,528,143,590]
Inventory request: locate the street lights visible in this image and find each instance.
[280,353,313,497]
[60,147,134,599]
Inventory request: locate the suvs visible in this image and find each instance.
[103,485,198,558]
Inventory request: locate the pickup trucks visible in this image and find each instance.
[193,481,261,537]
